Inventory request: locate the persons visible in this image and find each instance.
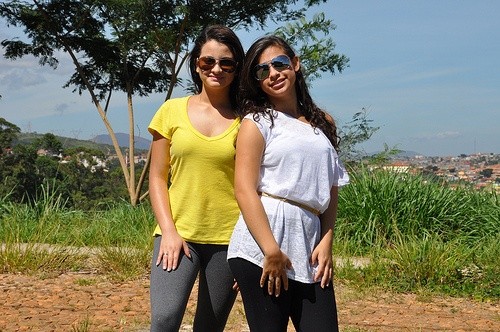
[149,25,244,332]
[226,36,350,332]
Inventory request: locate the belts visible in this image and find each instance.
[256,192,320,216]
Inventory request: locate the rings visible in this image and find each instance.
[268,279,274,283]
[163,253,168,255]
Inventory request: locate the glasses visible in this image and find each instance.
[197,56,238,73]
[253,54,290,81]
